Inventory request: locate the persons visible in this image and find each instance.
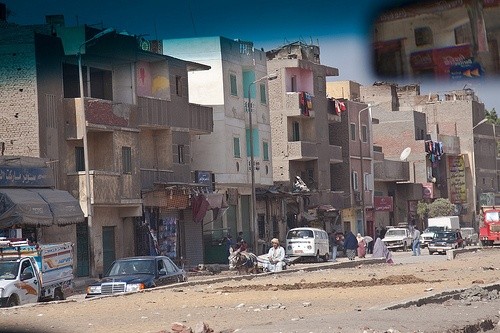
[412,226,421,256]
[455,229,462,239]
[329,230,338,262]
[373,238,388,258]
[380,226,386,239]
[344,231,358,259]
[356,233,367,258]
[268,238,286,271]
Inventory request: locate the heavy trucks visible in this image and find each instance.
[479,210,500,246]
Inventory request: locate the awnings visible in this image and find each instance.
[142,188,228,224]
[0,188,85,229]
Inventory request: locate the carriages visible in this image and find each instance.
[228,246,299,275]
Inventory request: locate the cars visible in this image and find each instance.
[382,228,413,252]
[84,254,188,298]
[460,228,478,246]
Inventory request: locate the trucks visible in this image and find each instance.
[0,236,74,307]
[418,216,460,250]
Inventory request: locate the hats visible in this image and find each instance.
[271,238,279,244]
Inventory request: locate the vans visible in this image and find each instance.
[285,227,330,262]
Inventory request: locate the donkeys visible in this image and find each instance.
[227,246,258,276]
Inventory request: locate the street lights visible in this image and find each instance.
[358,103,374,239]
[471,118,488,228]
[248,73,277,257]
[78,26,115,278]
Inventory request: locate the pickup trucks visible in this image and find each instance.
[427,230,463,255]
